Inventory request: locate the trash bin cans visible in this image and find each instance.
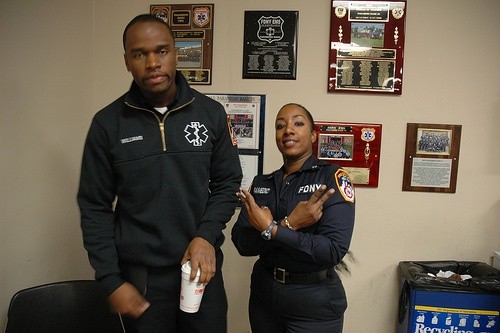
[398,261,500,333]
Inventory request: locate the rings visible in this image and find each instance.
[262,206,268,209]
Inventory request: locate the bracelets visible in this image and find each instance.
[284,216,293,231]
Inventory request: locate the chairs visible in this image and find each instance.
[5,280,117,333]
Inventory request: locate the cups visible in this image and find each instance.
[178,260,208,313]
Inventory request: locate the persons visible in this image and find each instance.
[231,103,355,333]
[243,128,251,137]
[76,14,243,333]
[418,133,449,152]
[321,140,351,159]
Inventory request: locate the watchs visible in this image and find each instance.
[262,221,277,241]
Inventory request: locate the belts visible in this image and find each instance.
[258,258,328,284]
